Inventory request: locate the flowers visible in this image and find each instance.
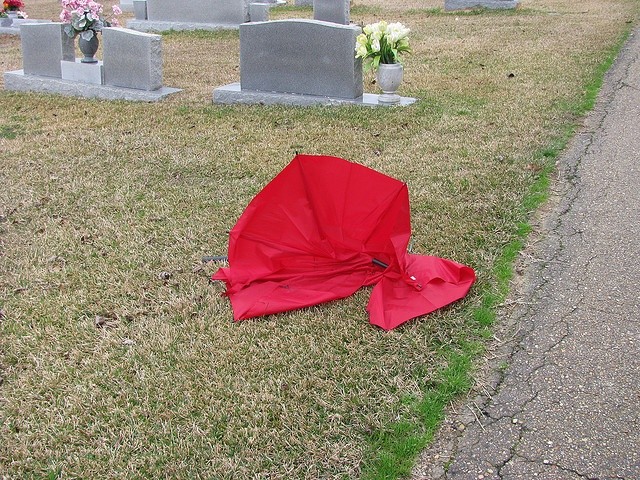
[352,19,414,69]
[58,1,123,41]
[0,0,24,17]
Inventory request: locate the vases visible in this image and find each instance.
[75,35,99,64]
[374,62,405,103]
[8,10,17,19]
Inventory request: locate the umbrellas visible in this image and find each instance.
[202,151,477,333]
